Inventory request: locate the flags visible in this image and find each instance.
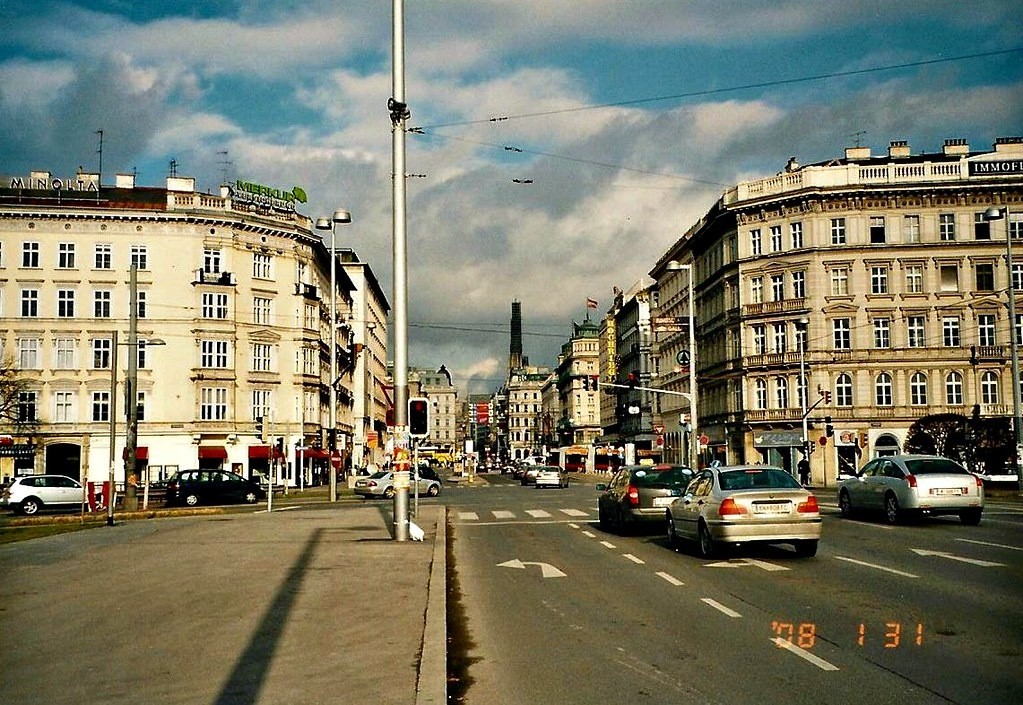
[587,297,597,308]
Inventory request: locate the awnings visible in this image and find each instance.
[123,447,149,459]
[249,446,270,458]
[198,446,227,459]
[565,447,661,456]
[297,447,330,458]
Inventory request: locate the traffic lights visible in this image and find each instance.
[582,373,598,392]
[327,426,336,451]
[811,441,815,453]
[275,435,285,458]
[409,398,430,438]
[346,342,357,370]
[315,429,326,450]
[825,391,832,405]
[254,415,267,443]
[860,432,868,448]
[827,425,834,437]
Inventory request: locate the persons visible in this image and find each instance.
[797,455,810,485]
[4,474,9,483]
[345,451,352,476]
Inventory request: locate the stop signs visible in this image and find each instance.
[331,453,342,468]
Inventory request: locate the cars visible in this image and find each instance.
[501,455,569,489]
[596,465,695,537]
[837,454,985,525]
[353,465,441,499]
[0,474,101,516]
[167,468,266,507]
[665,464,822,560]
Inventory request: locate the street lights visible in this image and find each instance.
[664,261,698,474]
[315,208,352,501]
[107,331,167,523]
[982,205,1023,501]
